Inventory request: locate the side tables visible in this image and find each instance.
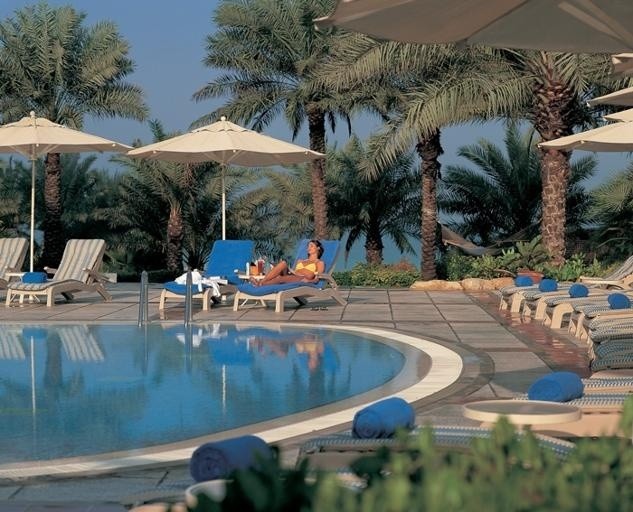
[5,271,40,305]
[237,274,267,307]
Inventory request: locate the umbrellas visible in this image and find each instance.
[611,54,633,80]
[0,111,135,302]
[312,0,633,54]
[604,107,633,122]
[536,123,633,152]
[586,87,633,108]
[126,116,326,305]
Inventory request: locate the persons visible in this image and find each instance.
[267,336,325,375]
[250,240,325,287]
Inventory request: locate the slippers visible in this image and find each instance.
[310,305,319,311]
[320,305,328,311]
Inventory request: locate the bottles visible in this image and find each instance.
[245,258,270,277]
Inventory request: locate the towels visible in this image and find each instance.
[189,435,272,487]
[22,272,47,284]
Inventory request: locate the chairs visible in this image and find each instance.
[233,239,348,313]
[123,467,389,512]
[159,240,259,311]
[0,236,29,289]
[5,238,113,307]
